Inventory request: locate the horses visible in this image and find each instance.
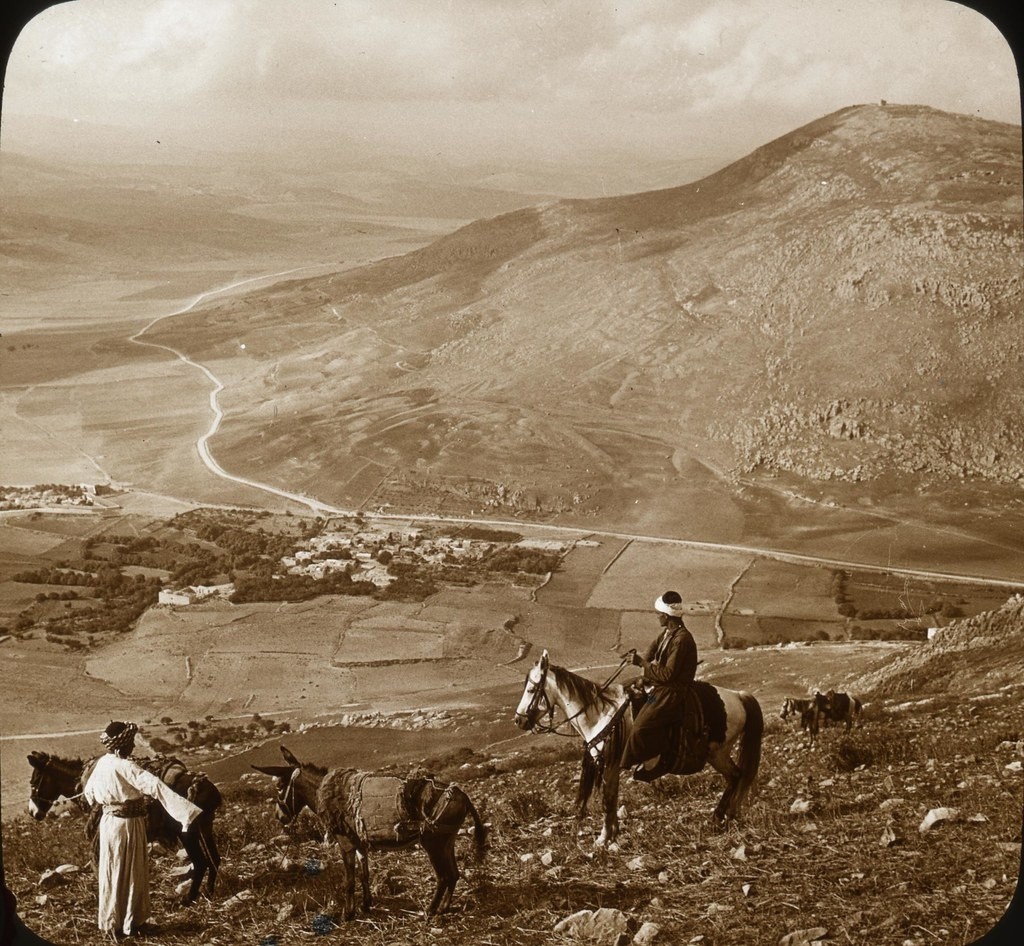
[513,649,765,849]
[249,745,487,926]
[780,693,864,749]
[26,750,223,907]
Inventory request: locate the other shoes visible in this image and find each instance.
[132,924,162,935]
[635,757,666,775]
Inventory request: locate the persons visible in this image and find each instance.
[84,722,203,939]
[620,592,698,780]
